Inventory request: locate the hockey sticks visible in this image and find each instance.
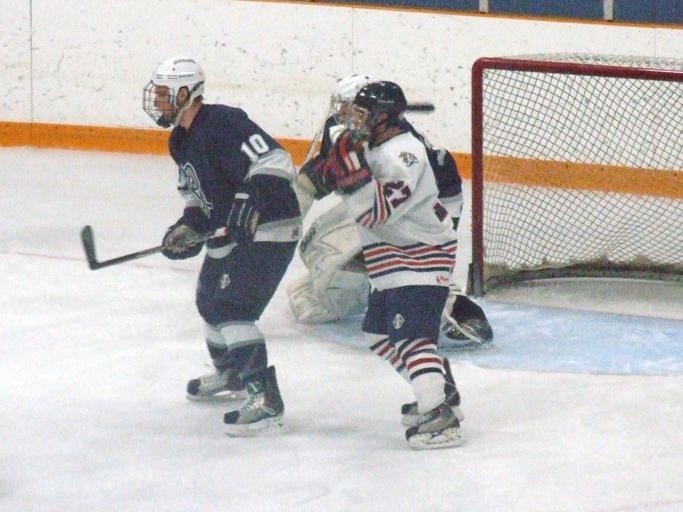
[81,224,226,270]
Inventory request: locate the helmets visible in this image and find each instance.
[142,56,203,128]
[329,73,408,142]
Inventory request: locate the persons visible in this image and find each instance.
[311,80,460,441]
[144,58,302,423]
[287,74,493,350]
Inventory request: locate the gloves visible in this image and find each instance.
[163,227,198,255]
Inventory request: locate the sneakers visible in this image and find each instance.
[401,374,460,416]
[406,404,460,440]
[223,366,284,425]
[187,368,245,393]
